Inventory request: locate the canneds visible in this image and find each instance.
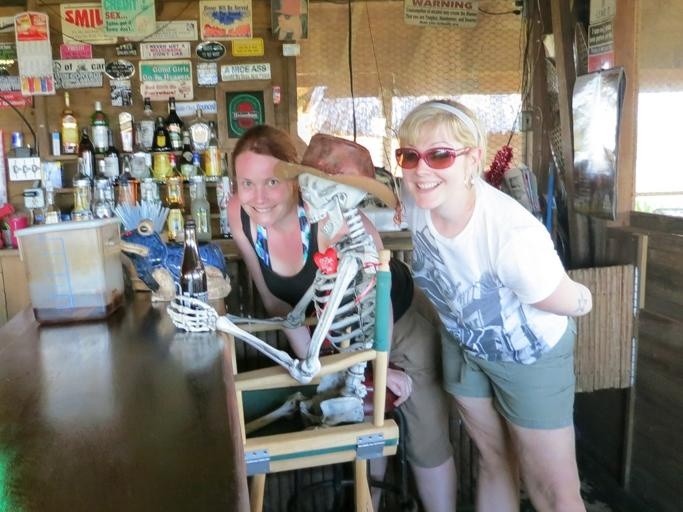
[11,131,23,150]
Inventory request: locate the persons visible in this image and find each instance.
[165,132,396,455]
[226,125,460,511]
[388,98,592,512]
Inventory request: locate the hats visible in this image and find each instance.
[272,134,399,212]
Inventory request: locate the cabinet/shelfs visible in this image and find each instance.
[32,94,226,244]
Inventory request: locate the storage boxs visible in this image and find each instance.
[12,219,126,324]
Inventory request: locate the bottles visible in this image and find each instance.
[218,176,233,238]
[153,116,172,152]
[179,130,195,177]
[116,176,134,209]
[189,108,211,151]
[60,91,80,155]
[72,158,92,220]
[138,98,156,152]
[89,100,109,155]
[45,193,60,224]
[191,183,213,244]
[166,183,186,241]
[104,129,120,181]
[204,120,223,177]
[165,96,183,151]
[165,154,184,209]
[189,152,207,202]
[215,158,232,206]
[176,219,209,333]
[76,128,95,181]
[92,160,114,218]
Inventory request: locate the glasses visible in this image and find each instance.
[395,148,471,169]
